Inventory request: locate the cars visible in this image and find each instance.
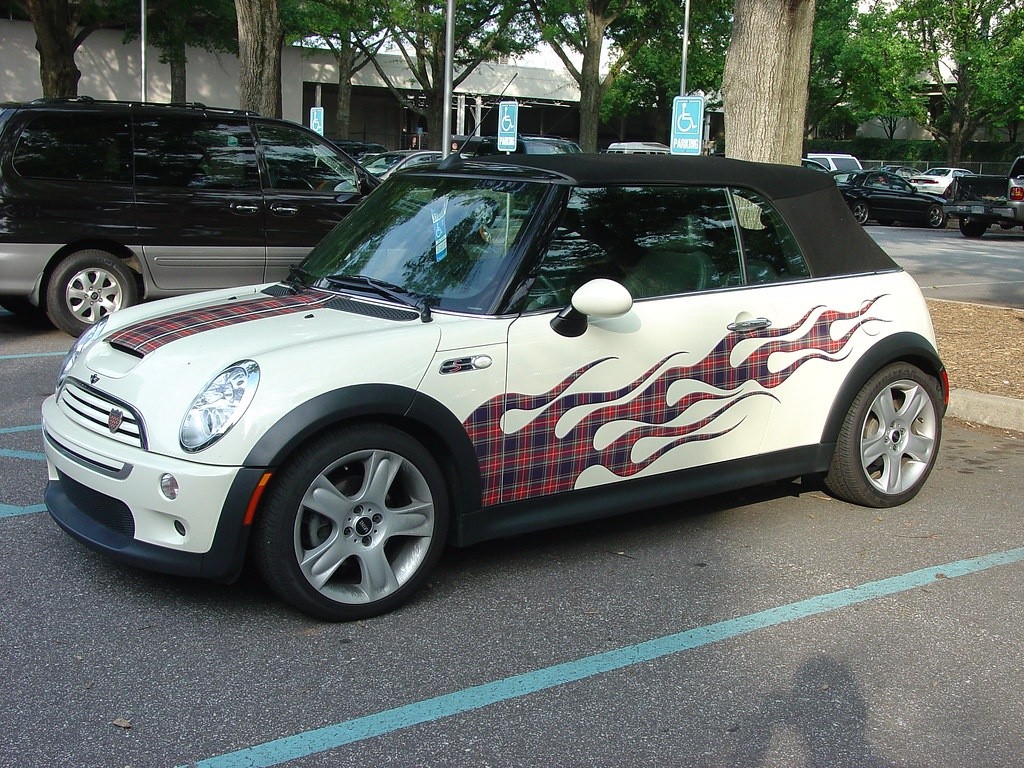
[867,165,922,180]
[908,167,974,197]
[36,153,952,623]
[326,138,389,166]
[828,171,950,228]
[607,141,670,154]
[360,150,470,182]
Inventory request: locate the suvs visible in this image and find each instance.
[476,135,583,156]
[0,95,388,338]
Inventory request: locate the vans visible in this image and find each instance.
[805,154,863,181]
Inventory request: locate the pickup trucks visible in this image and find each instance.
[942,156,1024,238]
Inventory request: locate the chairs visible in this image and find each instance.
[382,189,793,315]
